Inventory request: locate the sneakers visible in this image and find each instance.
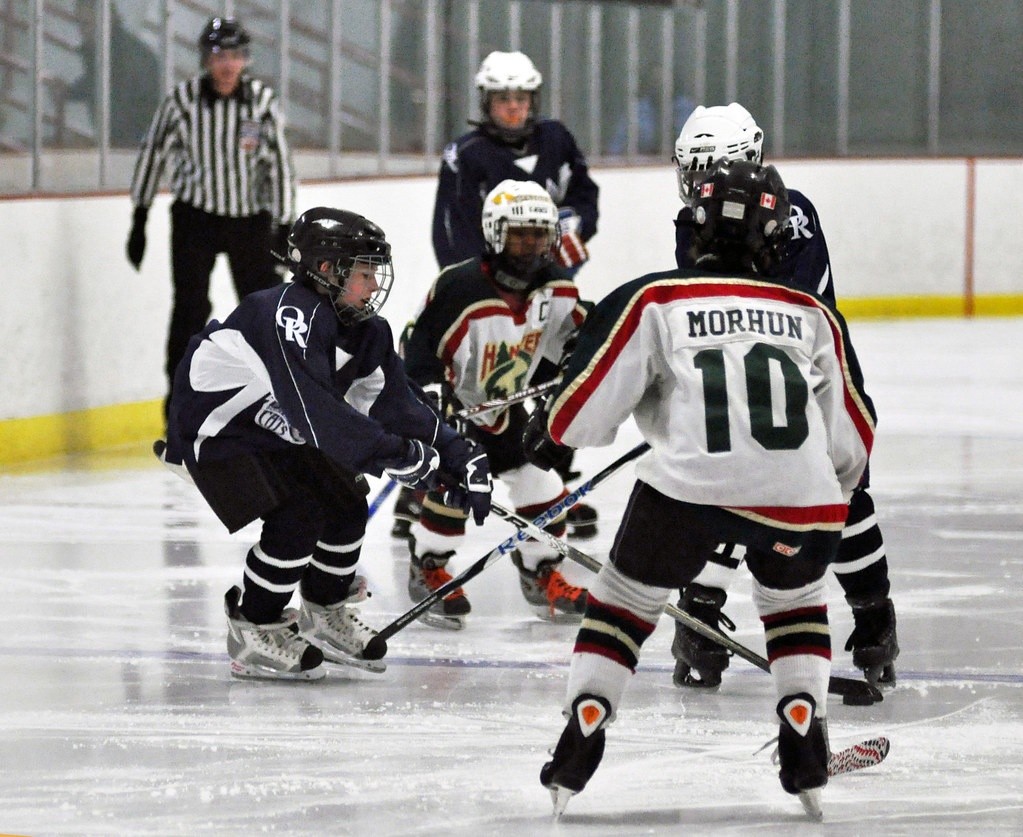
[296,575,387,674]
[511,546,590,623]
[407,533,472,632]
[839,580,899,689]
[541,692,612,819]
[672,582,736,690]
[753,691,832,820]
[221,586,327,682]
[516,492,600,540]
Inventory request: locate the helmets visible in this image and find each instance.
[482,178,560,261]
[287,208,394,327]
[670,101,764,203]
[474,51,544,96]
[694,156,792,279]
[199,17,251,54]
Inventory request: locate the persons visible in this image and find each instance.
[396,178,590,612]
[126,13,297,439]
[37,0,162,147]
[540,156,878,794]
[168,207,493,672]
[392,49,600,525]
[670,101,901,672]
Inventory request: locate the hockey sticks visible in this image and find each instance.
[437,470,886,704]
[367,480,396,518]
[458,375,565,418]
[378,440,650,641]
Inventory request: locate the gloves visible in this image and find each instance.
[547,207,590,282]
[523,413,580,471]
[446,443,493,525]
[127,205,150,269]
[384,439,447,492]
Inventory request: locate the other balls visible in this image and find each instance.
[843,685,875,706]
